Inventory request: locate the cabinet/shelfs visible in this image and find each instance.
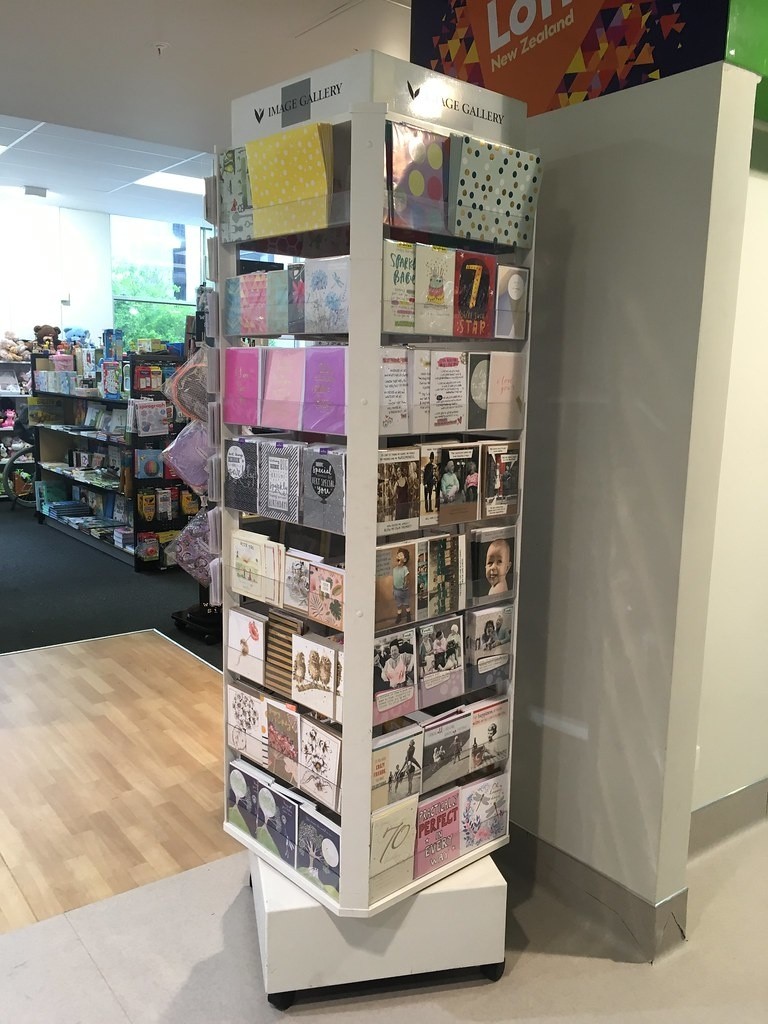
[1,45,545,1019]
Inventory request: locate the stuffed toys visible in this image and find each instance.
[0,325,96,362]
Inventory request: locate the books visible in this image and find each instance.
[218,116,544,905]
[26,398,134,555]
